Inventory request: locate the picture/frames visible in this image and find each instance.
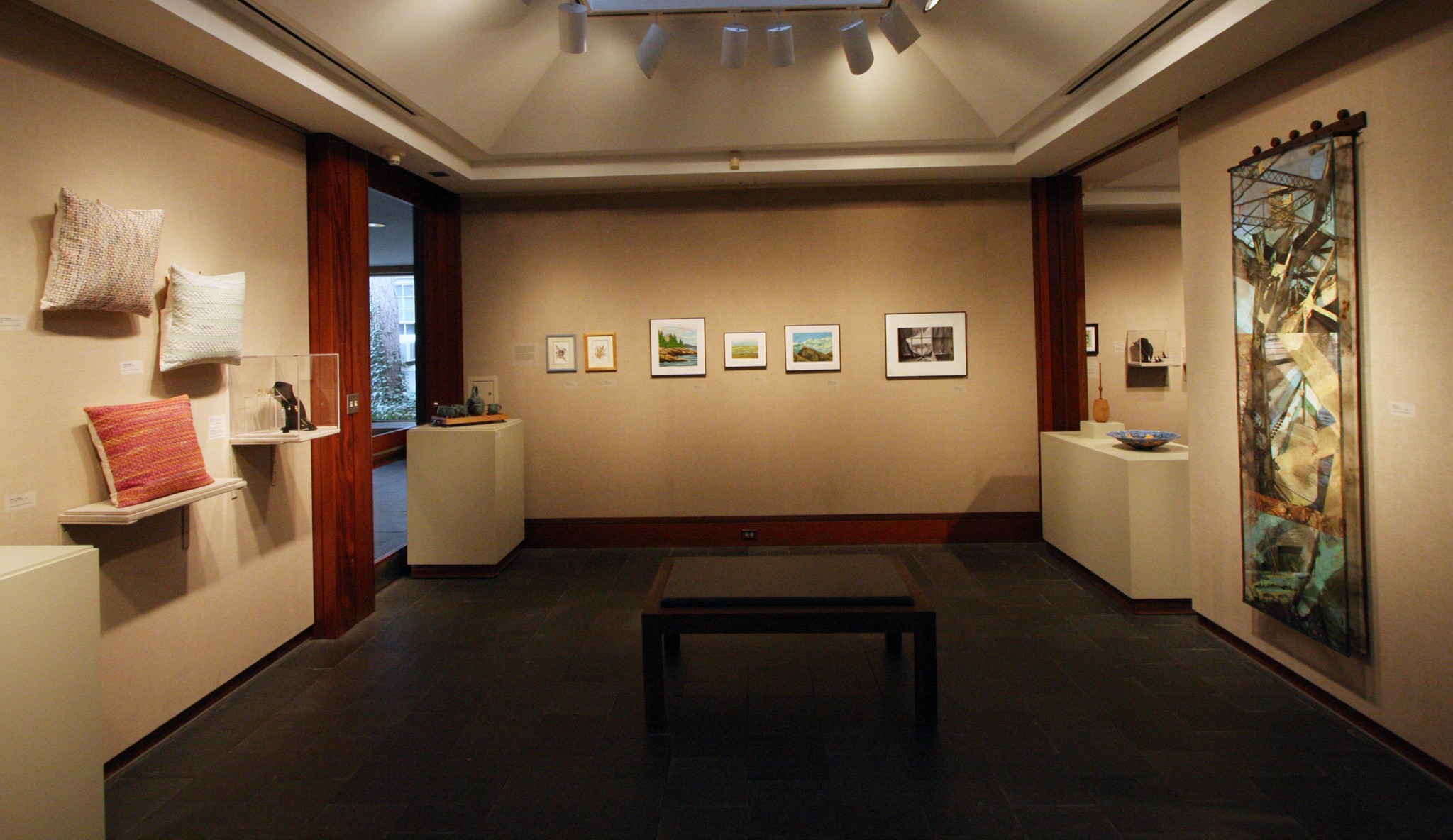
[650,316,708,377]
[883,311,969,378]
[582,331,617,373]
[784,323,841,372]
[1086,322,1103,355]
[546,333,579,373]
[723,331,768,368]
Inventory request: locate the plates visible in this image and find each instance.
[1106,430,1182,450]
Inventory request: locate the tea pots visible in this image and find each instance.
[466,386,484,416]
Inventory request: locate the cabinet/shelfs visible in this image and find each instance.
[62,426,345,543]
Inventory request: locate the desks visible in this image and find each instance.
[640,546,939,745]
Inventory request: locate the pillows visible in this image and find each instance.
[39,187,167,316]
[84,393,218,504]
[162,262,245,371]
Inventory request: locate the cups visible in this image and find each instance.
[436,405,468,418]
[488,403,502,414]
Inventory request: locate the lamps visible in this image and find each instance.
[556,0,939,74]
